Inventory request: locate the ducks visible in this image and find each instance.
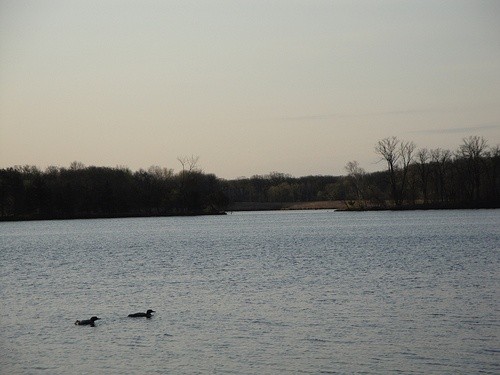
[74,316,101,325]
[128,309,156,317]
